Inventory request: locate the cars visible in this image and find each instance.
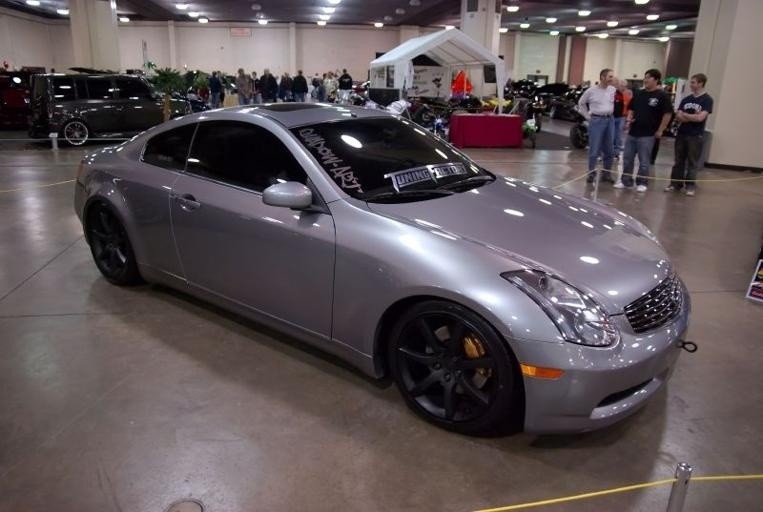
[349,79,371,97]
[498,76,684,135]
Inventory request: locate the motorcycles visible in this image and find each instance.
[414,90,540,151]
[566,105,630,152]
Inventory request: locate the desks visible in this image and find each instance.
[450,113,523,149]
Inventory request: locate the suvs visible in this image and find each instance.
[0,66,241,145]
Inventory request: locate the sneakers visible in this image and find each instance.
[685,189,696,197]
[601,173,614,183]
[612,182,634,189]
[635,185,647,192]
[586,176,594,183]
[663,185,681,193]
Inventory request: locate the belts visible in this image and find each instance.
[590,114,615,118]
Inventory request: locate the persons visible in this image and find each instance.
[215,70,225,103]
[576,65,714,193]
[208,71,220,109]
[236,68,352,104]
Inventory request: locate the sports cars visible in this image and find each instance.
[69,100,698,442]
